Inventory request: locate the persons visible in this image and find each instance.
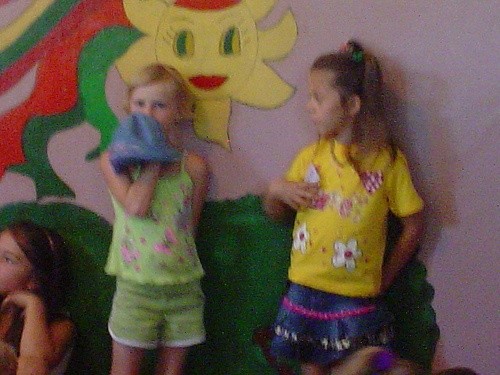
[261,39,426,375]
[1,218,79,375]
[98,63,212,375]
